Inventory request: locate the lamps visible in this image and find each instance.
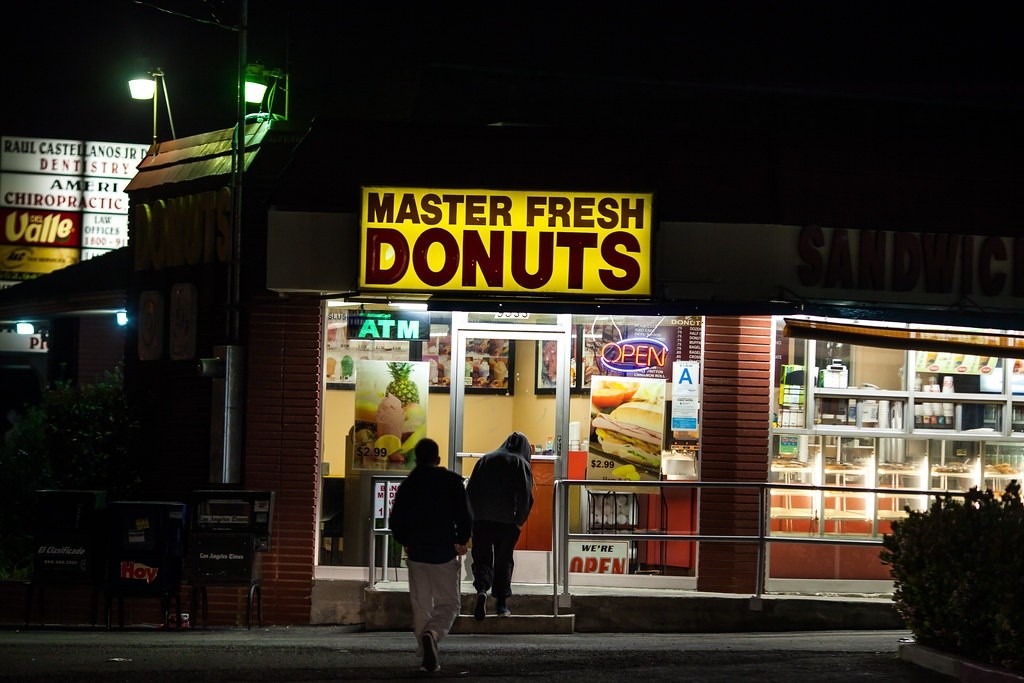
[244,63,270,122]
[128,71,164,157]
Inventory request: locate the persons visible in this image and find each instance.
[465,432,534,622]
[389,438,472,672]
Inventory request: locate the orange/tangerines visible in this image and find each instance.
[603,381,639,399]
[592,389,624,407]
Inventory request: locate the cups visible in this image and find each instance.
[465,356,507,385]
[326,325,346,344]
[340,354,355,379]
[374,411,404,444]
[427,356,451,384]
[327,357,337,375]
[914,376,957,393]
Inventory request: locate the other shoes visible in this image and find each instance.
[159,610,193,632]
[496,606,511,617]
[475,589,487,626]
[415,628,441,674]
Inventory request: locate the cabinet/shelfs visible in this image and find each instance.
[806,414,1024,459]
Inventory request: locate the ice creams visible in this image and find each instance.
[377,394,404,440]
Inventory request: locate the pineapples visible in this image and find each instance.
[386,362,419,408]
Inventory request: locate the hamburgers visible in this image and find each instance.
[592,402,662,468]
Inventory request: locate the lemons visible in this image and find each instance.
[375,435,400,459]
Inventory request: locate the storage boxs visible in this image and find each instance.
[779,364,819,406]
[779,409,805,427]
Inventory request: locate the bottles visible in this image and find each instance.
[545,435,554,450]
